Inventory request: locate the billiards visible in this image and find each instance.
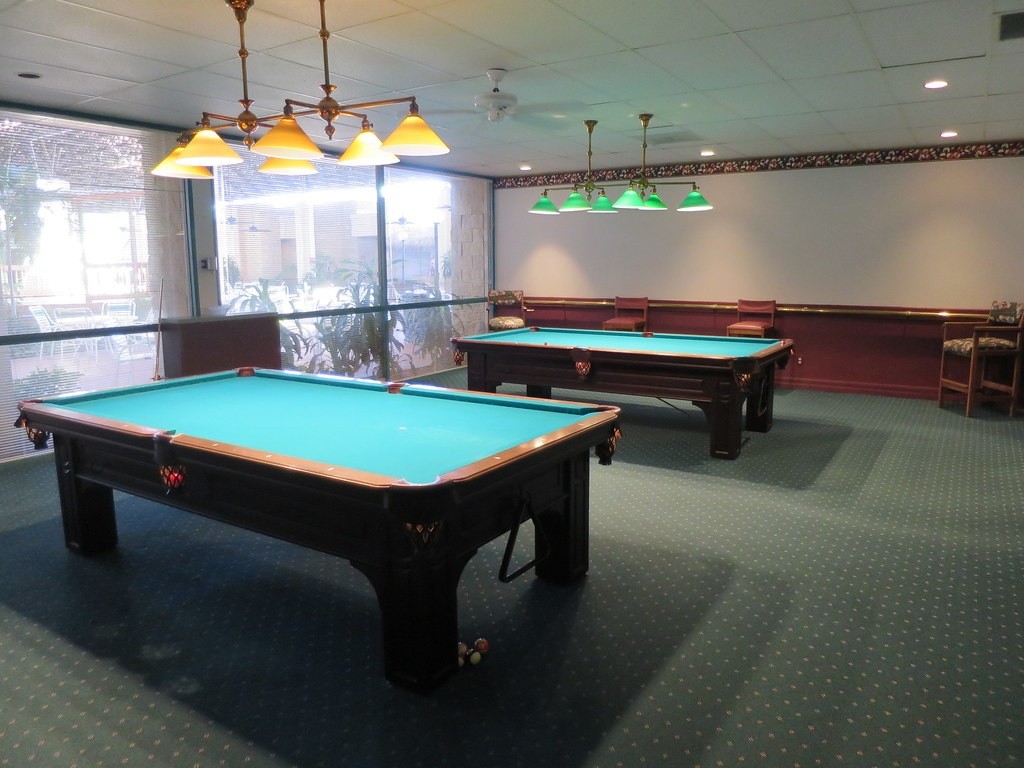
[457,637,491,668]
[167,472,183,487]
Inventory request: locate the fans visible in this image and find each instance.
[421,68,586,130]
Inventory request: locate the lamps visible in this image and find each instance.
[526,114,713,214]
[150,0,450,180]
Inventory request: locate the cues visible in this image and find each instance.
[153,277,164,382]
[656,397,691,417]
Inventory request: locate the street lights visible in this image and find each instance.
[424,202,452,297]
[387,217,415,302]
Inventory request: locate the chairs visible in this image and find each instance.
[602,296,649,332]
[486,289,527,332]
[940,300,1024,417]
[726,300,775,338]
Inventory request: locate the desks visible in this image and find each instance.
[450,326,794,459]
[15,370,623,695]
